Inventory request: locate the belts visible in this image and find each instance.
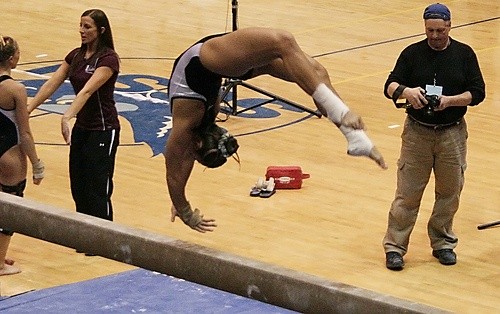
[408,115,463,131]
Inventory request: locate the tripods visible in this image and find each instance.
[218,0,322,119]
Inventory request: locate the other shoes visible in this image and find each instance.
[250,176,275,197]
[432,248,457,265]
[385,251,406,269]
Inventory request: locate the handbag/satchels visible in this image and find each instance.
[265,166,311,190]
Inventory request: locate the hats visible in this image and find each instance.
[423,2,452,21]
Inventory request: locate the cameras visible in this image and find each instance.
[419,93,441,114]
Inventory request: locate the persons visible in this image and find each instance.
[0,35,45,274]
[165,26,388,233]
[382,3,486,270]
[28,9,119,256]
